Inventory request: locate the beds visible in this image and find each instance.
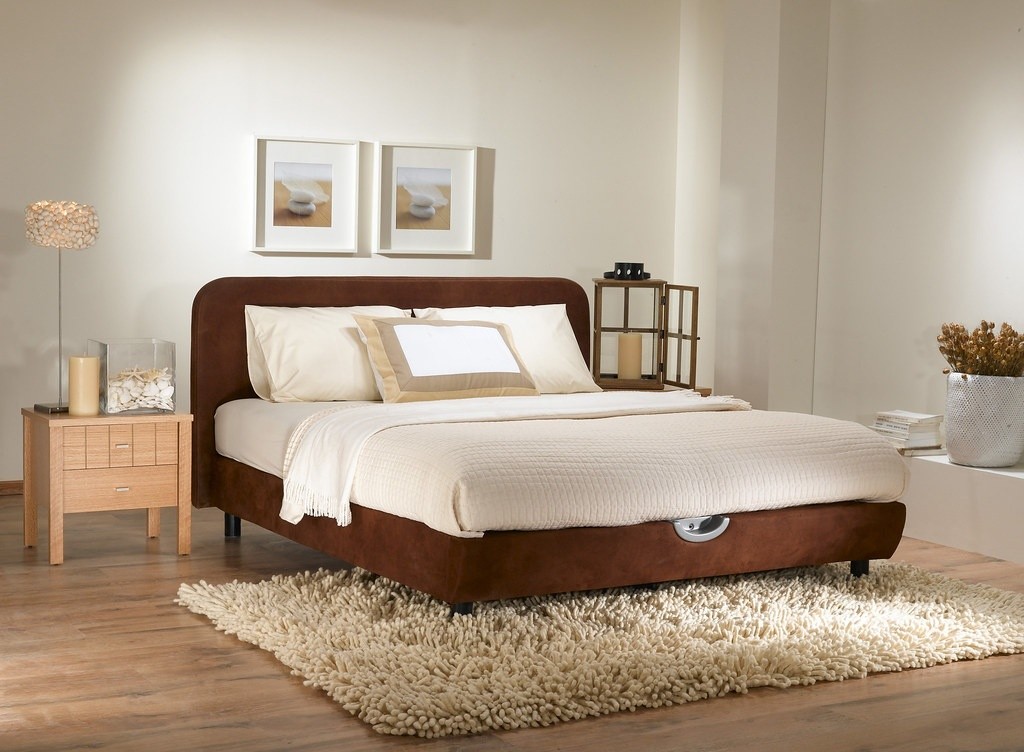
[192,274,910,621]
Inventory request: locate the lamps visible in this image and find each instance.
[24,200,101,414]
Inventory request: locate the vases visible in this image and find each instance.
[944,373,1024,469]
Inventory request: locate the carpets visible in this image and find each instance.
[177,563,1024,742]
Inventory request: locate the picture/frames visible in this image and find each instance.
[374,140,478,256]
[252,137,362,253]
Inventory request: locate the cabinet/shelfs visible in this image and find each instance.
[593,276,701,389]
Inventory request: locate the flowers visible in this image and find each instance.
[935,319,1024,380]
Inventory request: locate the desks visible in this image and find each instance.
[898,454,1024,565]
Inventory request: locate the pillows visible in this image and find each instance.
[415,303,602,396]
[354,312,541,403]
[244,303,412,405]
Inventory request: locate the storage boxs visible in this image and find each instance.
[87,336,177,414]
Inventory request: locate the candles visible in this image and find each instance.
[67,352,101,415]
[619,329,643,379]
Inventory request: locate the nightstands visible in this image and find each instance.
[650,384,712,397]
[21,406,197,567]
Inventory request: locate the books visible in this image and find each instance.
[868,409,947,448]
[896,449,947,457]
